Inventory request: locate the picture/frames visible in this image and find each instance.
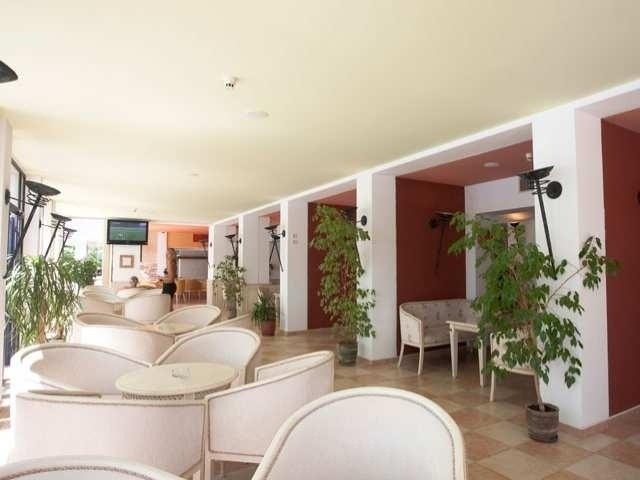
[119,255,134,268]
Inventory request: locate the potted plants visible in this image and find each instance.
[211,257,248,320]
[445,209,617,444]
[311,202,377,366]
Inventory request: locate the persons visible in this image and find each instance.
[156,247,180,302]
[126,276,138,288]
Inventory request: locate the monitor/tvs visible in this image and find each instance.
[106,219,148,244]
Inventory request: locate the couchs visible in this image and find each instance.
[489,324,536,403]
[400,297,479,374]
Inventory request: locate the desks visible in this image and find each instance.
[446,317,491,388]
